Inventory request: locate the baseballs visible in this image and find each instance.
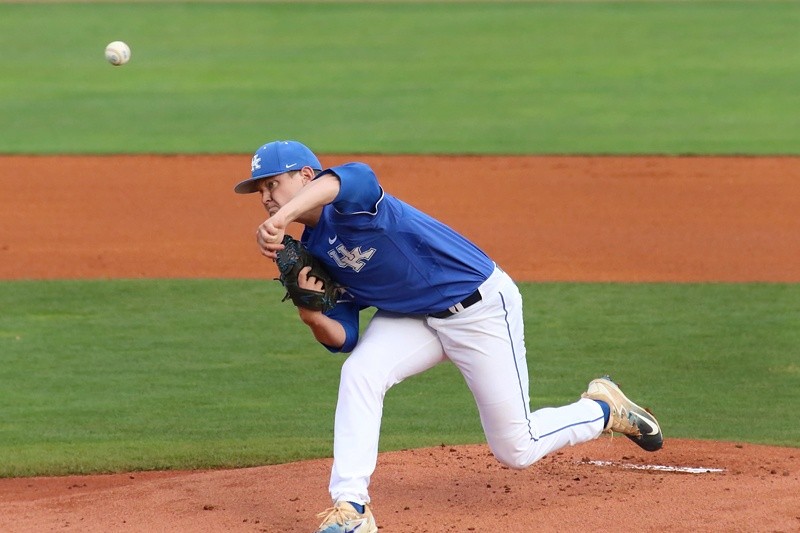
[105,41,131,65]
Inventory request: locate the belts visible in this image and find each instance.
[427,289,482,319]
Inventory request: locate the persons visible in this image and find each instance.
[232,139,664,533]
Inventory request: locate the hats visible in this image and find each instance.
[234,140,322,194]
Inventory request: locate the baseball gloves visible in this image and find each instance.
[276,234,339,311]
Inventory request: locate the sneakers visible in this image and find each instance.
[581,378,663,451]
[314,501,378,533]
